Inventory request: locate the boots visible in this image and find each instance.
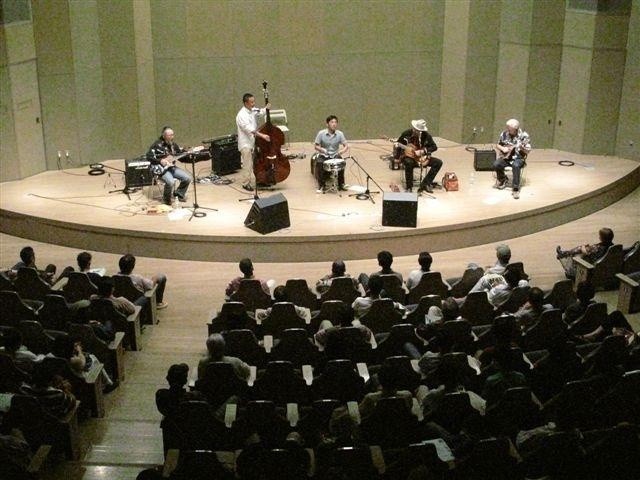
[556,246,583,259]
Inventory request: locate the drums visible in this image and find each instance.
[324,159,346,172]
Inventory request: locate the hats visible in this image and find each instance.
[496,243,510,256]
[411,119,427,132]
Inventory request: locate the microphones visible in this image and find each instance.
[347,155,352,159]
[419,132,422,144]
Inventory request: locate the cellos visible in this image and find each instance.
[253,80,289,186]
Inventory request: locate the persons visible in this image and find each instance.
[145,127,191,205]
[314,114,349,195]
[397,119,444,196]
[491,118,532,200]
[235,93,273,192]
[135,228,639,480]
[1,246,168,480]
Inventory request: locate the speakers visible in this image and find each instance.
[383,192,418,227]
[474,150,497,171]
[209,137,241,176]
[125,158,157,188]
[244,193,290,235]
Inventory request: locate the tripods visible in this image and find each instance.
[182,161,218,221]
[417,143,436,200]
[352,158,384,204]
[109,172,137,200]
[239,145,260,201]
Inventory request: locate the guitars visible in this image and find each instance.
[498,139,528,159]
[151,146,202,175]
[384,135,430,166]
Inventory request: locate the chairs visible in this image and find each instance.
[152,240,640,480]
[149,162,178,204]
[398,155,428,189]
[492,148,528,190]
[265,108,293,148]
[0,267,157,480]
